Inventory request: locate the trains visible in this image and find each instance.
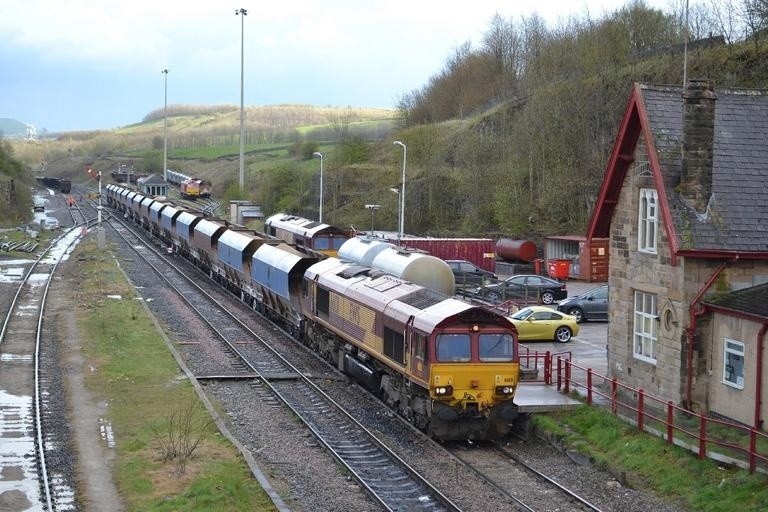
[36,177,71,193]
[166,170,212,200]
[108,183,520,442]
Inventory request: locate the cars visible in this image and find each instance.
[557,287,609,324]
[506,306,579,343]
[474,275,567,305]
[446,259,497,285]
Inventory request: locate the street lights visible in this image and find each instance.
[236,8,247,192]
[390,187,401,246]
[313,153,323,224]
[161,69,168,182]
[393,140,406,237]
